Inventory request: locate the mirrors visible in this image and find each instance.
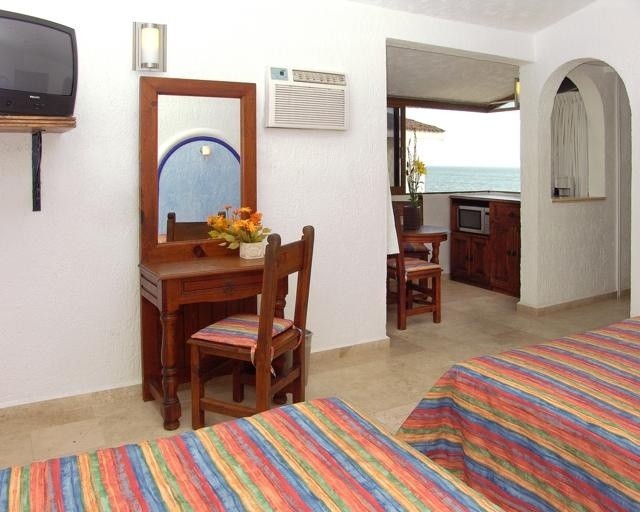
[138,76,257,263]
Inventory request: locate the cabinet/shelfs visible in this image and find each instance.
[449,196,520,299]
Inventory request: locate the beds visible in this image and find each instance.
[0,396,505,512]
[395,315,640,512]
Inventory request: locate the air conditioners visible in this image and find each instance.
[265,66,348,133]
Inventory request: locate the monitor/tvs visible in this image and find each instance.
[0,9,79,117]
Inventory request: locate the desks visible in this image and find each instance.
[141,255,288,431]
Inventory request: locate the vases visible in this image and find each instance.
[404,207,420,230]
[240,242,266,259]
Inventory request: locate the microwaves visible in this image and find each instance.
[455,205,490,236]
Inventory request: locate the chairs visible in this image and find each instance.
[392,200,429,299]
[388,202,443,330]
[188,226,314,432]
[167,212,207,241]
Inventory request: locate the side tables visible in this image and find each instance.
[401,225,448,265]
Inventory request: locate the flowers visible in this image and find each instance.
[404,130,427,207]
[219,206,271,249]
[207,204,231,239]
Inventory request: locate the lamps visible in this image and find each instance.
[132,22,167,72]
[200,146,210,155]
